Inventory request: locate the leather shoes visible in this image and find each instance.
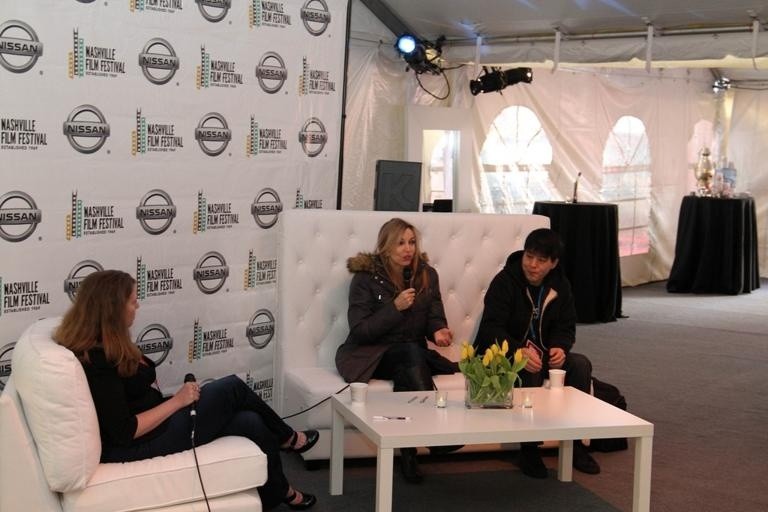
[519,447,548,480]
[572,444,602,477]
[399,448,425,484]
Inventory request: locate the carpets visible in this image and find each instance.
[259,467,625,512]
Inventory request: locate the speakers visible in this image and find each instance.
[373,159,423,211]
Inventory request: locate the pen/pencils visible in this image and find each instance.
[372,416,411,420]
[420,396,428,403]
[407,396,418,404]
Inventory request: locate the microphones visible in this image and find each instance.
[568,172,583,203]
[402,266,413,317]
[183,372,200,438]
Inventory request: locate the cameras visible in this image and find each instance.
[394,35,447,77]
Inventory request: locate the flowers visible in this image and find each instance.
[457,337,531,405]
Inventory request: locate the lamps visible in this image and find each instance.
[399,35,430,73]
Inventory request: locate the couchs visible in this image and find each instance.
[271,211,551,462]
[1,317,269,512]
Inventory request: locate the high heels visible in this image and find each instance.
[278,487,318,509]
[281,429,320,452]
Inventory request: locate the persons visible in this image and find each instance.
[471,228,602,478]
[50,270,320,509]
[335,218,455,484]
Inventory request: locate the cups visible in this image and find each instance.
[349,382,369,405]
[549,369,566,390]
[435,391,449,408]
[523,391,533,409]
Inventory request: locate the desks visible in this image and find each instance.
[664,192,763,296]
[532,200,624,326]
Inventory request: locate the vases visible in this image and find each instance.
[464,377,514,409]
